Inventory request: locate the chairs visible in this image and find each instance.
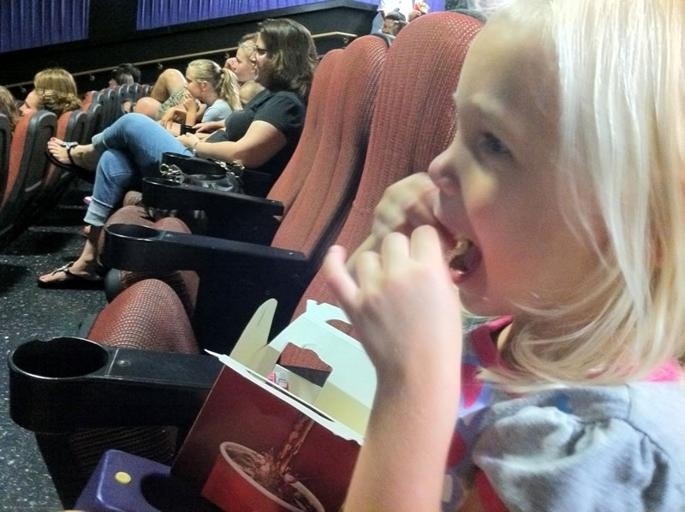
[1,8,490,512]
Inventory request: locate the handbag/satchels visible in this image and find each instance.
[147,163,246,222]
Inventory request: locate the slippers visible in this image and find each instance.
[37,261,103,291]
[45,140,95,183]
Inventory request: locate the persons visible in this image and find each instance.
[1,1,684,510]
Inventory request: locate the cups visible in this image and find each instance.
[199,441,324,512]
